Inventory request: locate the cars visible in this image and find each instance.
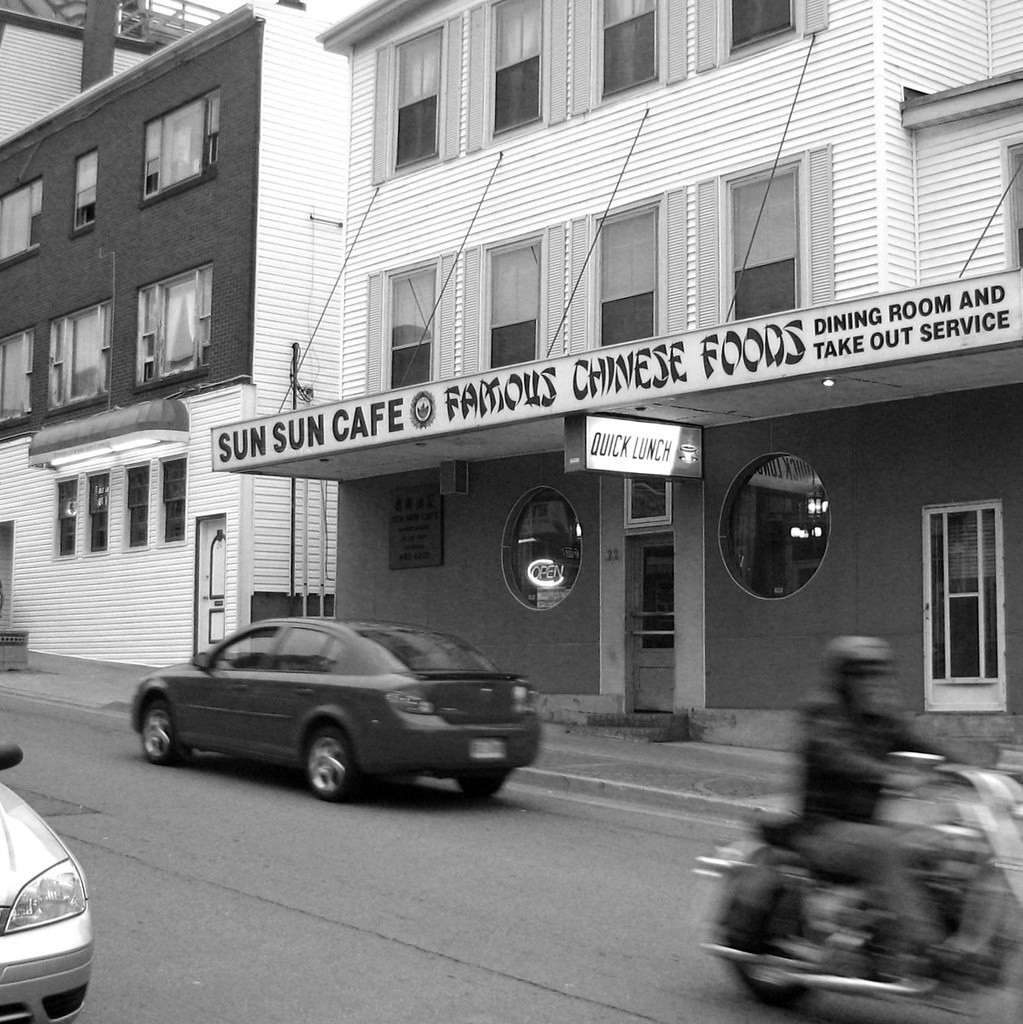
[0,743,93,1024]
[130,617,540,804]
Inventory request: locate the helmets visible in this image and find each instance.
[829,635,893,687]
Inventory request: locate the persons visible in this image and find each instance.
[791,636,969,981]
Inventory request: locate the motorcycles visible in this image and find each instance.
[690,752,1023,1011]
